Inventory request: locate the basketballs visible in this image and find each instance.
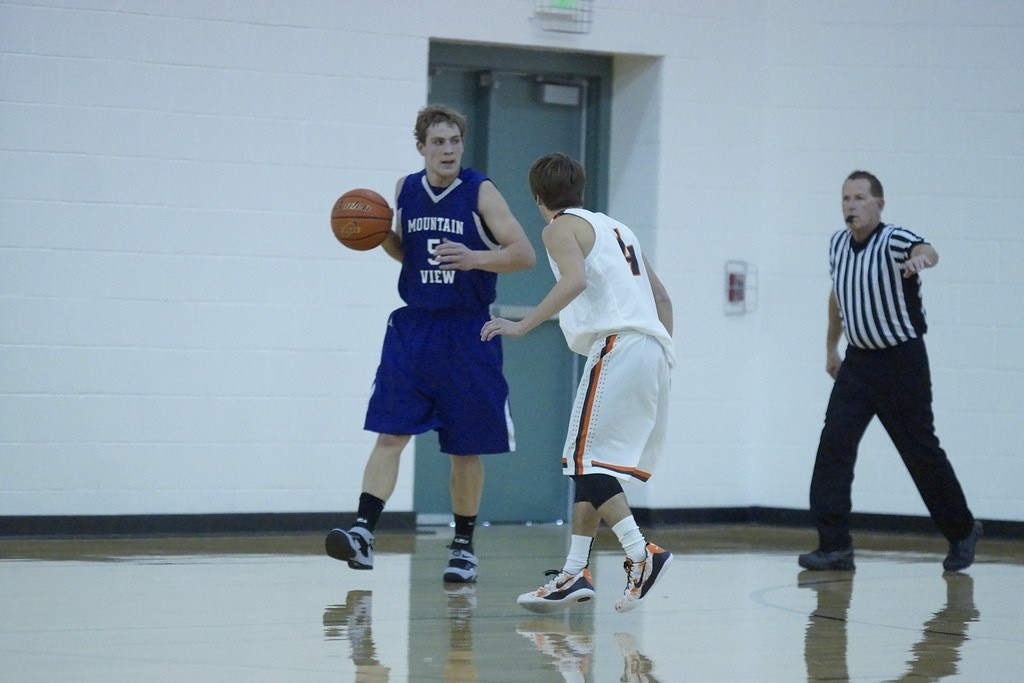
[330,188,393,252]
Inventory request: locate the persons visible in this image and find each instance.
[799,172,983,572]
[326,106,537,582]
[480,152,674,614]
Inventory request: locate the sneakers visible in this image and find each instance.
[443,549,479,584]
[321,590,373,631]
[516,619,595,673]
[942,571,980,618]
[615,540,675,613]
[325,526,375,570]
[518,569,595,614]
[615,632,660,683]
[943,521,984,571]
[798,539,857,571]
[798,578,854,609]
[447,595,477,630]
